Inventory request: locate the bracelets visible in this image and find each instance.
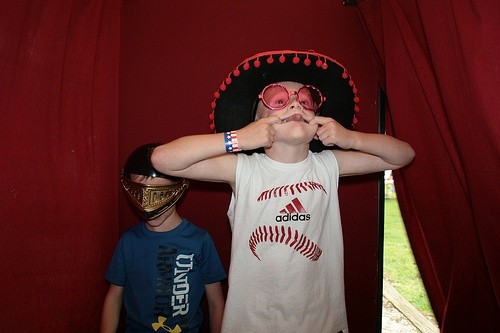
[224,130,238,153]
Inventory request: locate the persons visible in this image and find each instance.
[149,49,416,333]
[97,141,229,333]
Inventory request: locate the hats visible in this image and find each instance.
[209,51,359,153]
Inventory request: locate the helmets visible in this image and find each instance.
[120,142,188,220]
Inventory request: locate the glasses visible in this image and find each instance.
[257,83,326,110]
